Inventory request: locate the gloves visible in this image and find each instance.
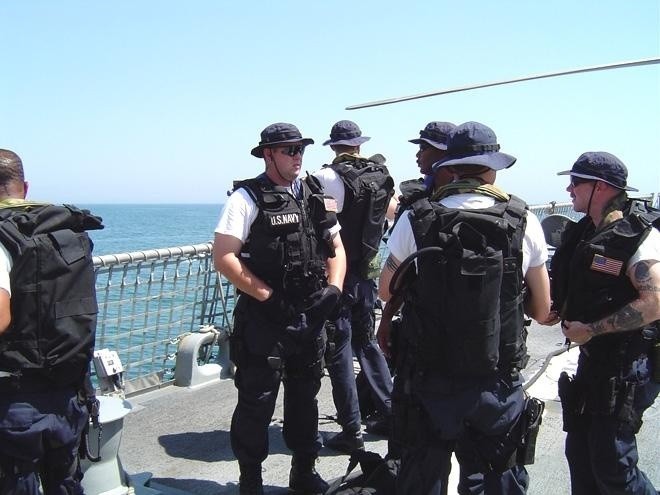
[261,285,342,347]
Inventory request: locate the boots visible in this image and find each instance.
[289,451,330,495]
[238,460,264,495]
[366,407,391,436]
[323,422,365,453]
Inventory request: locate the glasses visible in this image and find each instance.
[281,145,305,157]
[571,176,597,188]
[420,144,431,152]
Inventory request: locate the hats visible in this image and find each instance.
[557,152,639,192]
[251,123,314,157]
[322,120,372,147]
[432,121,517,173]
[408,121,457,151]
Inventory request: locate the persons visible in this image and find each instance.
[307,120,397,454]
[214,123,348,495]
[407,123,456,194]
[0,149,95,495]
[550,151,659,495]
[380,123,554,495]
